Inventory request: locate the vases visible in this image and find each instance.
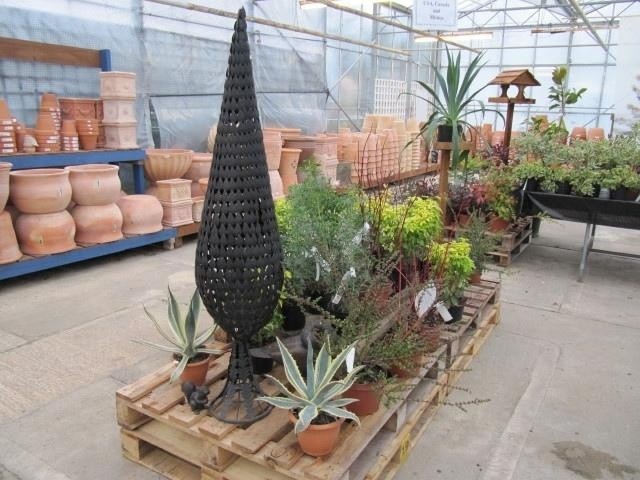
[0,123,219,265]
[260,114,438,202]
[1,68,140,155]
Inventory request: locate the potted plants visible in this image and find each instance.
[510,63,640,205]
[253,156,519,458]
[131,280,230,394]
[400,39,509,176]
